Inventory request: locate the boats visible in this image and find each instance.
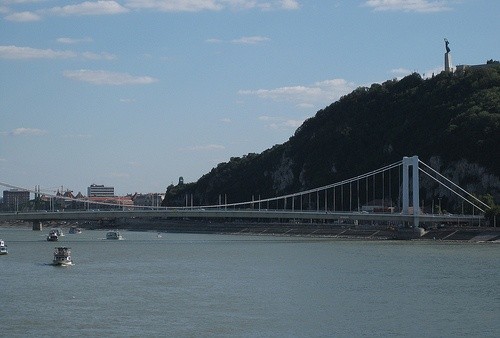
[53,247,75,266]
[47,230,64,241]
[385,225,424,239]
[106,231,122,239]
[69,226,82,234]
[0,240,8,255]
